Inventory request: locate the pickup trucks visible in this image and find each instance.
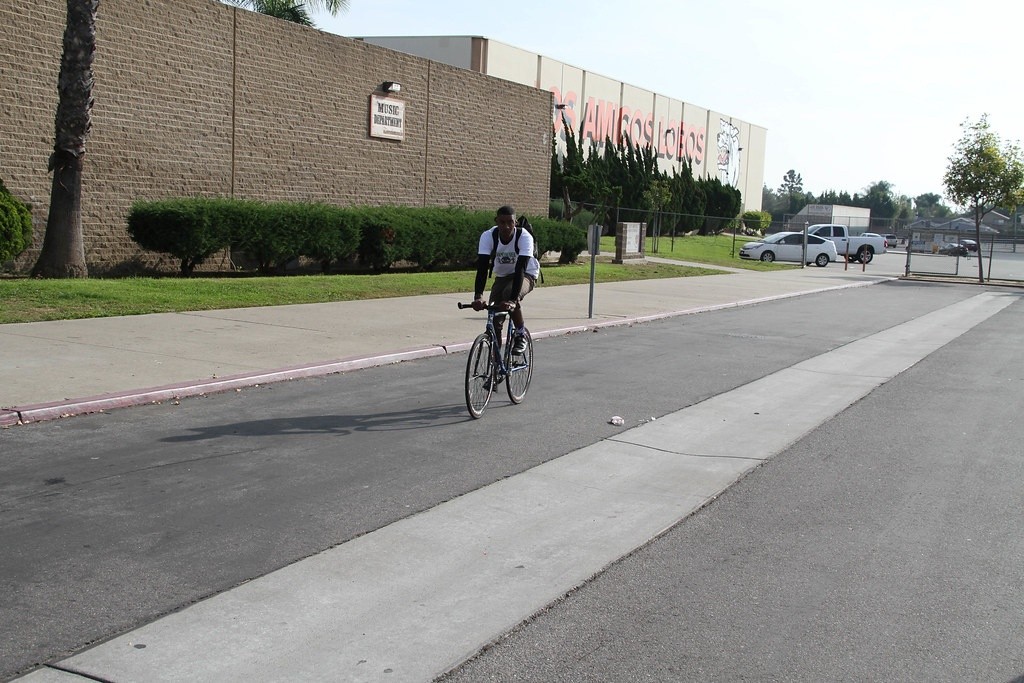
[800,224,889,264]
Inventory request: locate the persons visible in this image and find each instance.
[472,206,541,392]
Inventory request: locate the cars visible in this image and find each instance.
[960,240,978,252]
[939,243,968,257]
[739,231,839,267]
[860,232,880,237]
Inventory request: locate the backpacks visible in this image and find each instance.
[489,215,538,279]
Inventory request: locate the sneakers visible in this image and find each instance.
[483,380,497,391]
[512,336,528,354]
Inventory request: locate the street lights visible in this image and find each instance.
[1013,213,1023,253]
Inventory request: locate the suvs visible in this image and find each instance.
[879,234,898,248]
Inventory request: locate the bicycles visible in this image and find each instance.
[458,302,535,421]
